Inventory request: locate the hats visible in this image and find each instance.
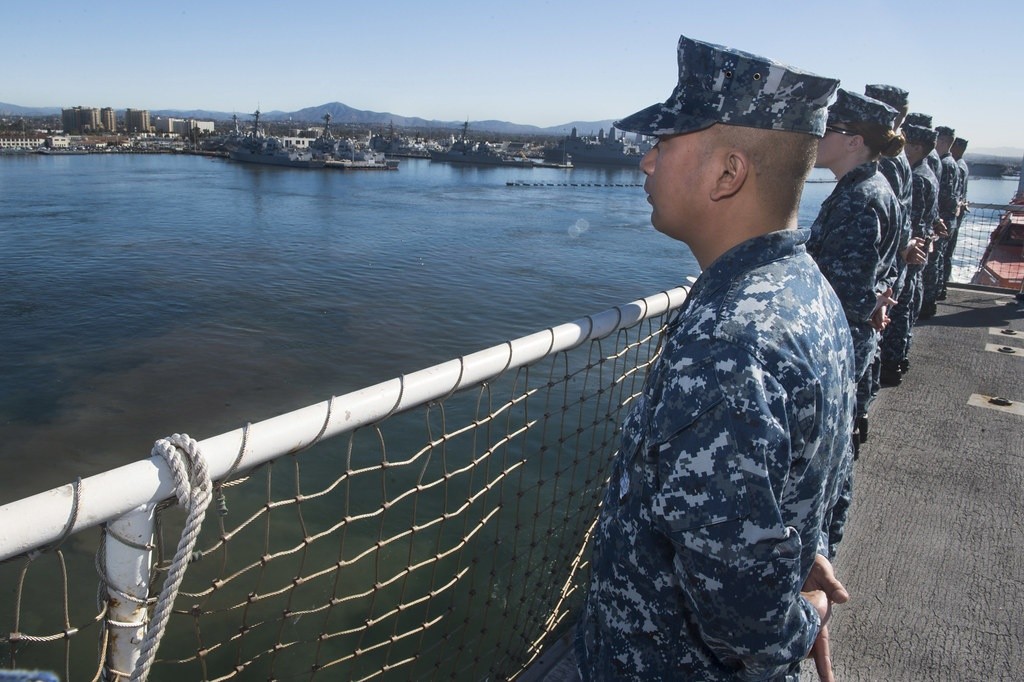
[899,113,932,127]
[901,124,938,141]
[612,35,840,138]
[827,88,900,130]
[864,84,909,106]
[935,126,955,136]
[954,137,968,147]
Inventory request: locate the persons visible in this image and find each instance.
[806,85,970,460]
[571,35,856,682]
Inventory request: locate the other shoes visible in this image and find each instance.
[852,428,860,460]
[859,417,869,440]
[936,290,947,300]
[919,312,930,319]
[880,370,902,386]
[900,358,908,371]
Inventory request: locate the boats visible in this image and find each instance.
[224,101,645,170]
[969,190,1024,290]
